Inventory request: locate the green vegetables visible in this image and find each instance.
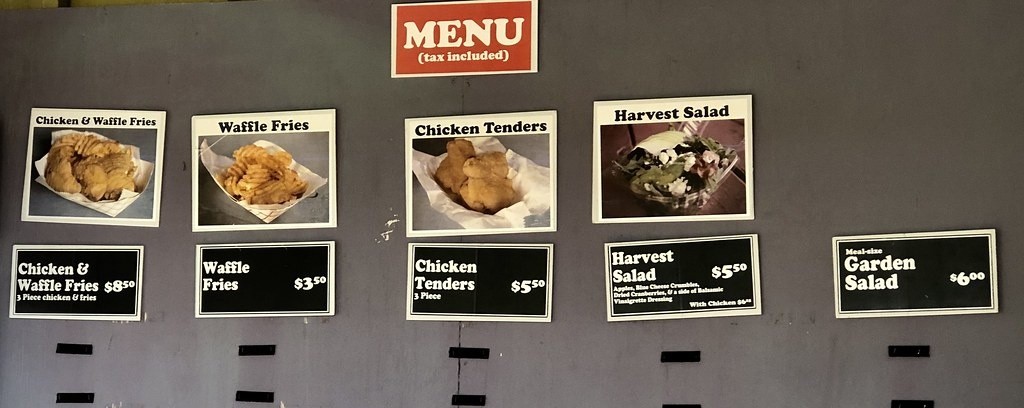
[611,134,731,200]
